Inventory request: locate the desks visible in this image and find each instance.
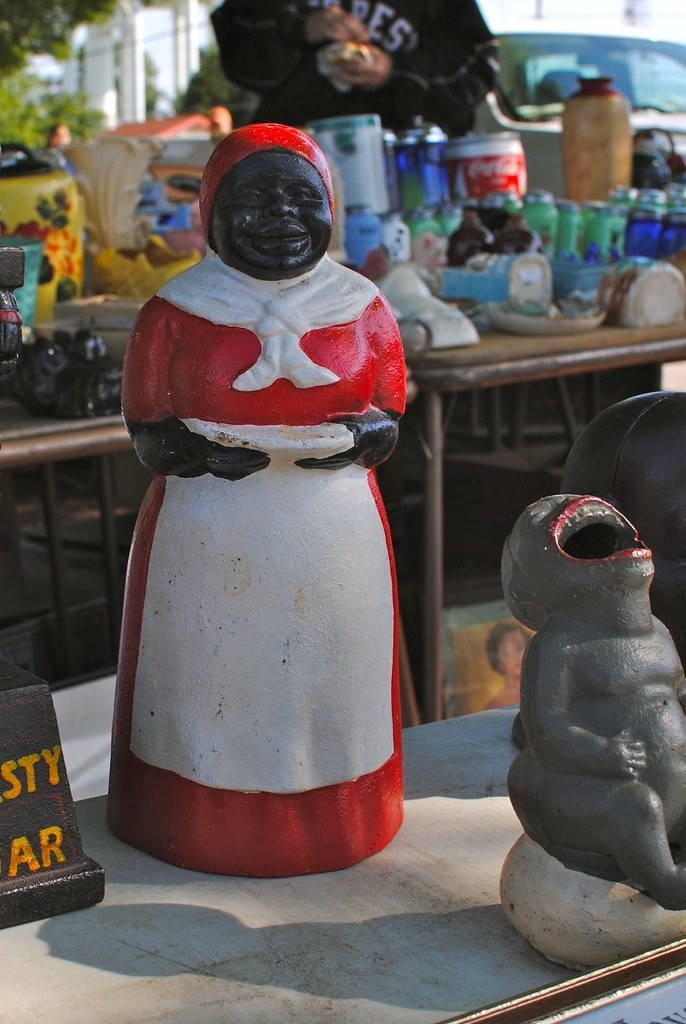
[0,398,134,685]
[385,247,685,726]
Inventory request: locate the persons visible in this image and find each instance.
[46,124,74,152]
[104,124,408,879]
[210,1,503,139]
[499,495,686,911]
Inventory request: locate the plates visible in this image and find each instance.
[491,305,606,334]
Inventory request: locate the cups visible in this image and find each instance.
[306,115,390,218]
[0,236,43,329]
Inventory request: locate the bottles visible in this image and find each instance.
[343,173,686,317]
[563,75,631,199]
